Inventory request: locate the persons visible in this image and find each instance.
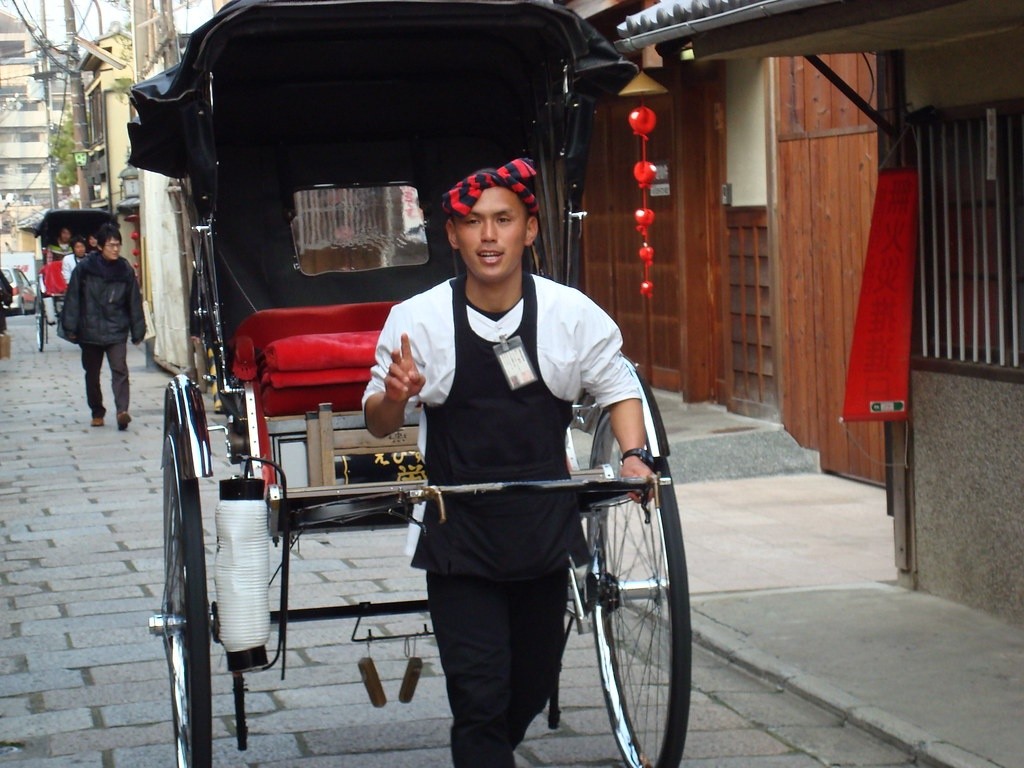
[0,269,12,334]
[64,225,146,429]
[361,160,657,768]
[47,225,102,289]
[511,376,520,388]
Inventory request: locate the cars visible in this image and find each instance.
[0,267,36,316]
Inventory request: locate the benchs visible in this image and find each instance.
[40,260,71,295]
[232,299,403,416]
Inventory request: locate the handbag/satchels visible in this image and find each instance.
[58,308,80,340]
[0,332,11,359]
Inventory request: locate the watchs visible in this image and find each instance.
[622,448,654,469]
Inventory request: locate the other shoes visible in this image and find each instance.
[92,418,104,426]
[117,411,131,430]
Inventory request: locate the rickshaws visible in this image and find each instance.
[32,208,121,351]
[148,0,693,768]
